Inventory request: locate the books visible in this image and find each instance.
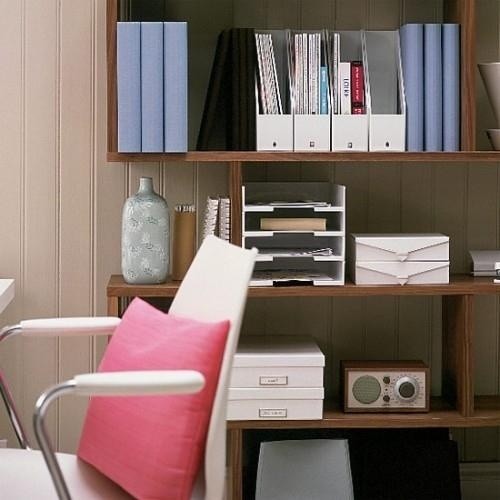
[255,33,365,113]
[117,21,188,155]
[259,217,328,231]
[398,24,461,154]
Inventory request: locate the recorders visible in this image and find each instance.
[341,359,430,413]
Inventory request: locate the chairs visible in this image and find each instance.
[1,235,258,499]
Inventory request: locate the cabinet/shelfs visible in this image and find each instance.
[105,1,500,499]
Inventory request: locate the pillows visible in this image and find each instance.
[75,297,231,499]
